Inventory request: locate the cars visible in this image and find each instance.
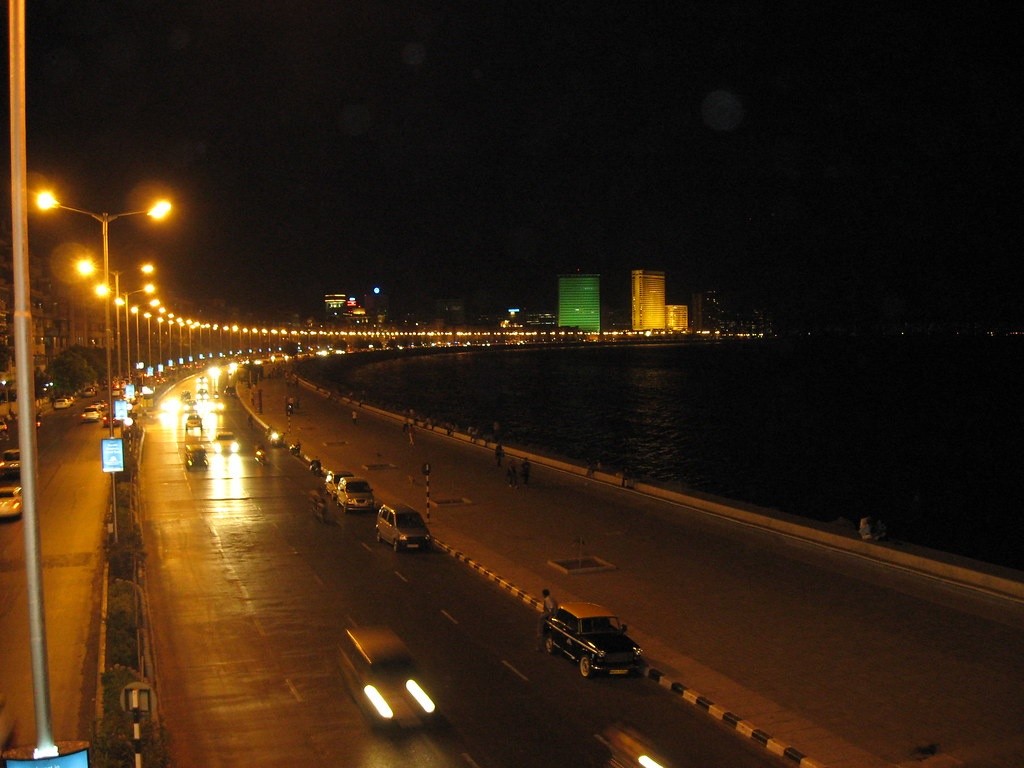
[223,384,237,400]
[79,407,103,423]
[0,448,23,523]
[53,398,71,410]
[543,599,645,679]
[102,412,121,429]
[83,387,97,398]
[89,402,106,411]
[185,414,203,435]
[331,625,442,734]
[112,387,124,397]
[0,420,9,442]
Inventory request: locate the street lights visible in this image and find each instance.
[73,255,156,375]
[90,281,158,376]
[159,307,327,369]
[33,186,174,437]
[114,296,160,363]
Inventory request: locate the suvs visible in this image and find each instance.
[184,445,209,471]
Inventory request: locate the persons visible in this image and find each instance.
[285,373,298,414]
[402,406,492,446]
[496,440,503,464]
[507,463,518,488]
[402,415,409,435]
[521,457,530,484]
[352,409,357,424]
[536,589,558,639]
[409,424,415,445]
[493,419,499,444]
[592,460,600,473]
[622,466,633,488]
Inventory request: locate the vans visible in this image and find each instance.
[374,503,433,554]
[325,470,354,502]
[336,477,375,514]
